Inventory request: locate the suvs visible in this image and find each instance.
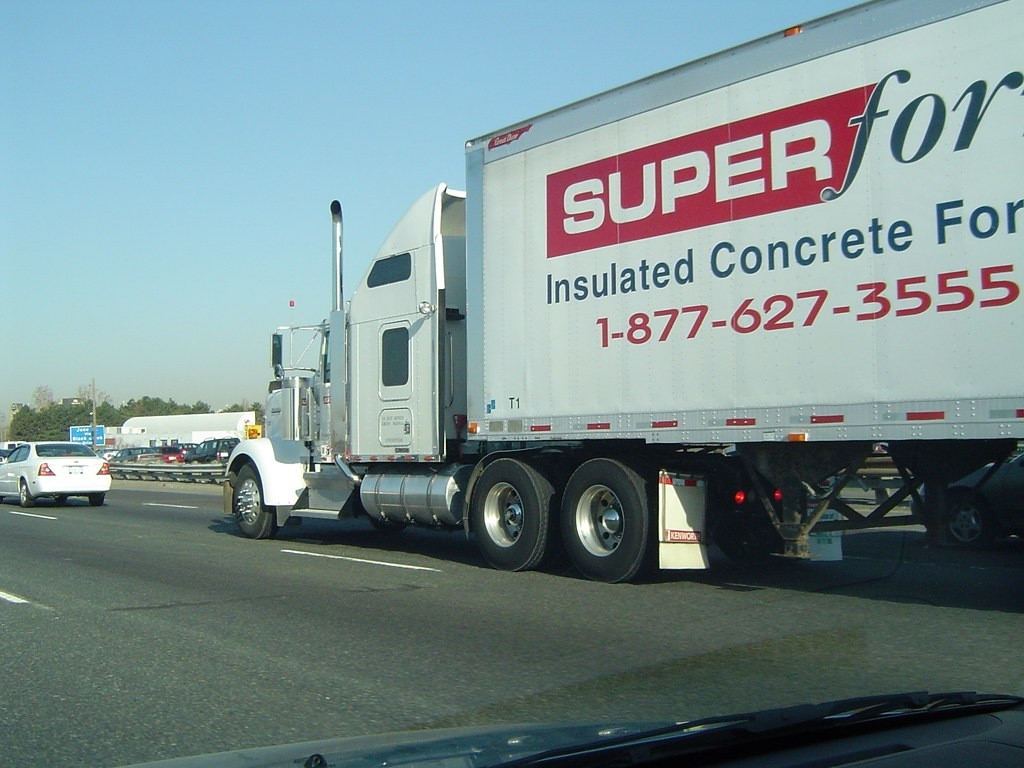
[182,438,240,465]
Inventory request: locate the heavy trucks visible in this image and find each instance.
[222,0,1023,584]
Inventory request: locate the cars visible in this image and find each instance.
[0,440,112,508]
[96,441,199,462]
